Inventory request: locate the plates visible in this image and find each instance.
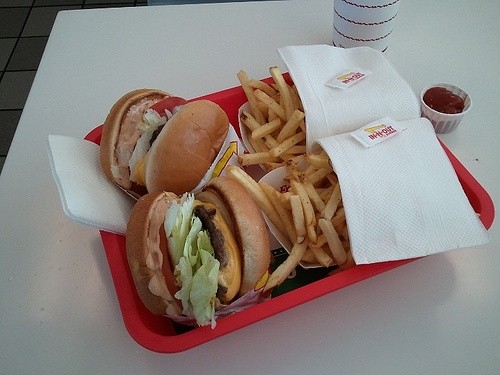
[83,72,495,353]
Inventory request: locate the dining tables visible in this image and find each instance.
[1,1,500,375]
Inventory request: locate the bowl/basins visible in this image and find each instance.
[421,82,471,136]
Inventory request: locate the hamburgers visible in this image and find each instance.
[124,175,272,331]
[98,88,247,198]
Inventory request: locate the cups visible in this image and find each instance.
[331,0,400,56]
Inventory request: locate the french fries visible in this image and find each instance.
[226,149,355,297]
[236,66,309,171]
